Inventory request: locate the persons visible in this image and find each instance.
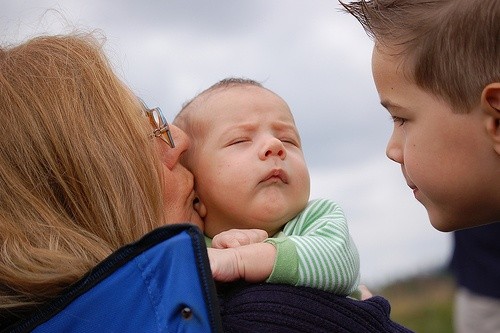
[0,31,419,333]
[171,77,372,302]
[337,0,500,333]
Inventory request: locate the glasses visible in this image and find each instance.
[148,107,176,150]
